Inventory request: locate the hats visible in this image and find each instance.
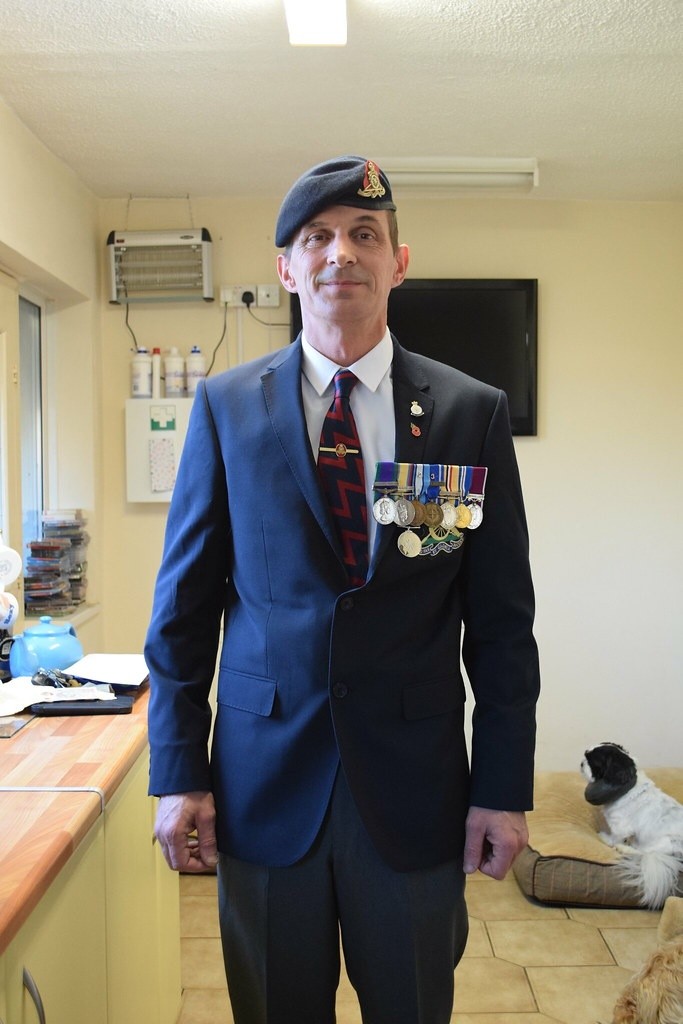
[274,152,398,250]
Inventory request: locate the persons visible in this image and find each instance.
[145,156,540,1024]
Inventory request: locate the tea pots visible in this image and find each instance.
[10,616,82,678]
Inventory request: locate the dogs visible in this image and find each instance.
[581,741,683,910]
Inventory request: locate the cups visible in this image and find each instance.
[0,545,22,585]
[0,592,19,629]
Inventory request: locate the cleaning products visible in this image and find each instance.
[130,345,206,399]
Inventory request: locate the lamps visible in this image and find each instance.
[365,156,538,188]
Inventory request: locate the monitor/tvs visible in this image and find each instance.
[289,277,539,436]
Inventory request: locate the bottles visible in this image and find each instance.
[186,346,206,398]
[165,348,184,398]
[132,347,161,398]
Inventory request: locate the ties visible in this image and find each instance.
[315,366,374,590]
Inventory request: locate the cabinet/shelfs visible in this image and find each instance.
[103,740,183,1023]
[0,811,104,1023]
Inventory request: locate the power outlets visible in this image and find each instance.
[219,284,256,308]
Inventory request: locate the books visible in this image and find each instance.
[24,507,89,615]
